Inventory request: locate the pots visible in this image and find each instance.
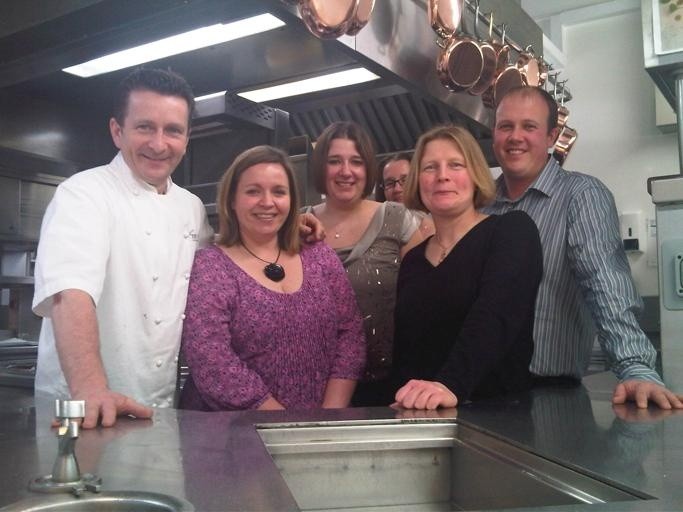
[429,0,462,47]
[482,24,522,109]
[468,0,497,97]
[514,44,539,87]
[345,0,375,36]
[484,11,510,81]
[298,0,357,39]
[553,71,569,131]
[551,80,578,167]
[437,2,483,93]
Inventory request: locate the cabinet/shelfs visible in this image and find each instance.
[0,166,67,243]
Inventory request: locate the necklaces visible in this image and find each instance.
[239,236,285,281]
[434,214,480,263]
[324,202,367,238]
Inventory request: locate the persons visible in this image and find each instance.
[375,150,430,214]
[297,121,424,407]
[32,67,327,428]
[390,126,543,410]
[179,145,369,410]
[476,86,683,410]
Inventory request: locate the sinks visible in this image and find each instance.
[0,491,197,512]
[255,417,657,512]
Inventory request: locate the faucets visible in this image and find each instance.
[50,396,87,481]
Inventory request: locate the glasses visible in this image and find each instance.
[378,175,408,191]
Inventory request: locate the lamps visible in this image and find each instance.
[232,61,383,107]
[61,8,286,79]
